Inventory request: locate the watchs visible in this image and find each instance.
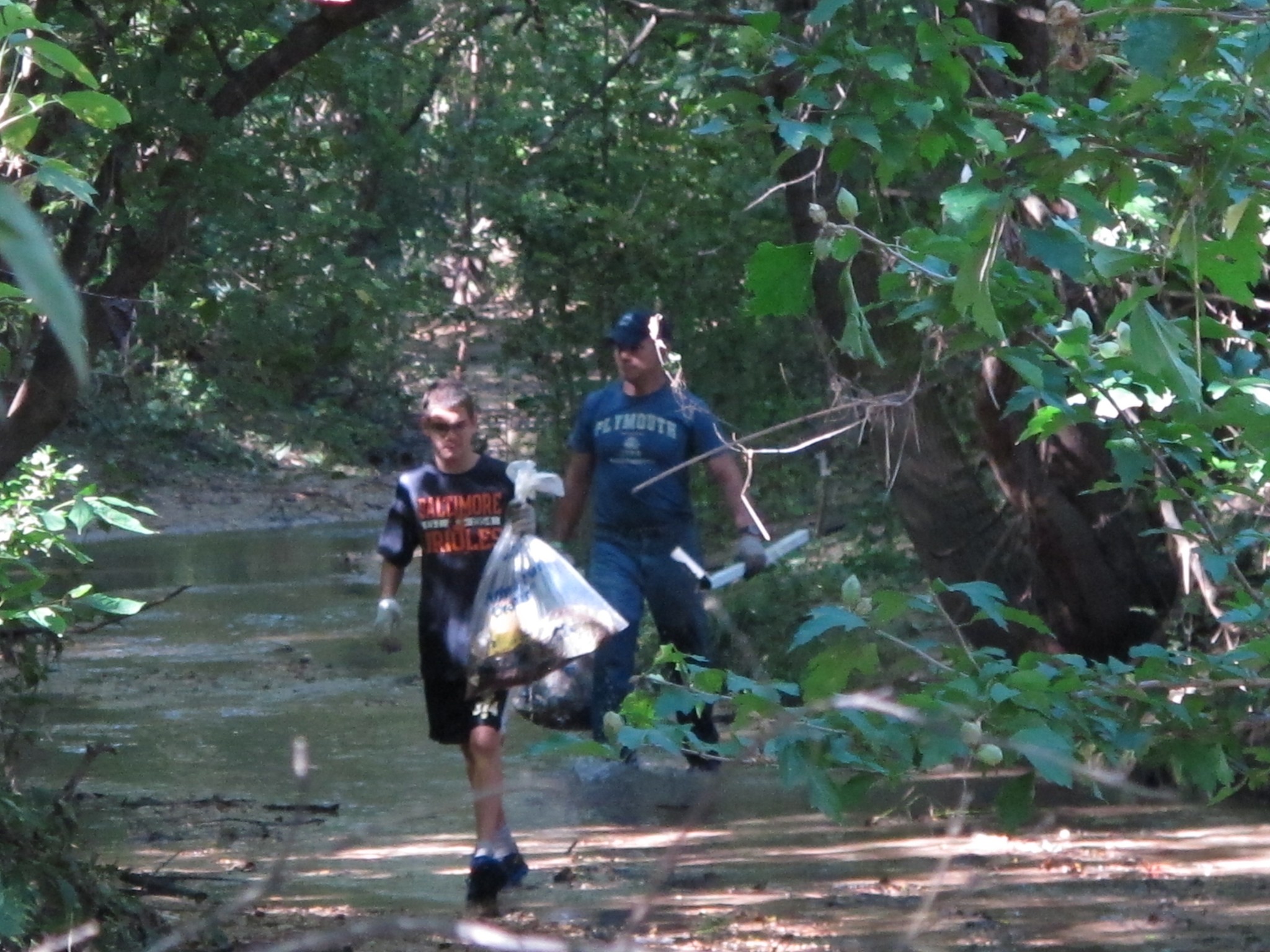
[738,524,764,543]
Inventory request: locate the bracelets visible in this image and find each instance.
[378,598,399,612]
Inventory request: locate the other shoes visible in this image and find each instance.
[612,741,639,772]
[678,718,723,769]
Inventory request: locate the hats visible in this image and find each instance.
[603,309,670,352]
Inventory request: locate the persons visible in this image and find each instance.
[551,309,766,775]
[373,380,539,936]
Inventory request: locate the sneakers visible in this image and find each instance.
[499,851,528,891]
[464,856,505,918]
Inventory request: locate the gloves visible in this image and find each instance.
[373,599,403,652]
[735,539,766,582]
[508,499,536,534]
